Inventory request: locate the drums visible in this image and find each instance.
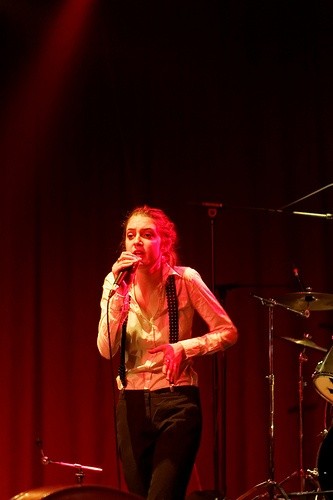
[311,344,333,404]
[12,481,149,500]
[233,481,288,500]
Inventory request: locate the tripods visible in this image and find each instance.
[236,285,325,500]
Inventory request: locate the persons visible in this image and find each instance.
[97,208,239,500]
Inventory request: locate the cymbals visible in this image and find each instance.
[280,336,329,352]
[276,290,333,311]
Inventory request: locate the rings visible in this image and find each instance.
[118,258,121,263]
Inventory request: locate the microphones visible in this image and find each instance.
[108,268,130,298]
